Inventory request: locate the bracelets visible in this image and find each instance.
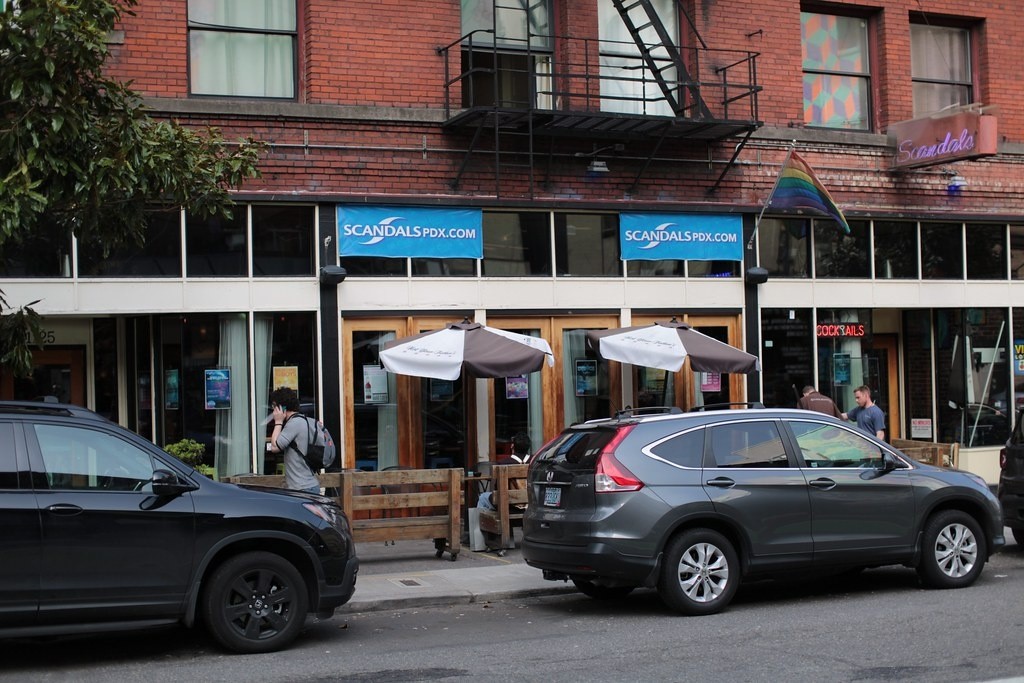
[275,424,283,426]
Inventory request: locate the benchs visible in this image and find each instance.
[227,463,527,567]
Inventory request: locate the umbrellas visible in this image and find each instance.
[587,317,760,413]
[379,316,555,547]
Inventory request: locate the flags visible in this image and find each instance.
[769,150,851,233]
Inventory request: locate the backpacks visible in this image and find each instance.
[508,453,531,509]
[292,413,336,470]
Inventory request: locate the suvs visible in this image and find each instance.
[519,401,1008,617]
[0,398,360,653]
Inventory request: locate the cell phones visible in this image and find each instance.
[278,404,286,413]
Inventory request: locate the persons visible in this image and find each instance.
[476,434,534,514]
[841,386,886,441]
[270,386,321,495]
[797,386,844,421]
